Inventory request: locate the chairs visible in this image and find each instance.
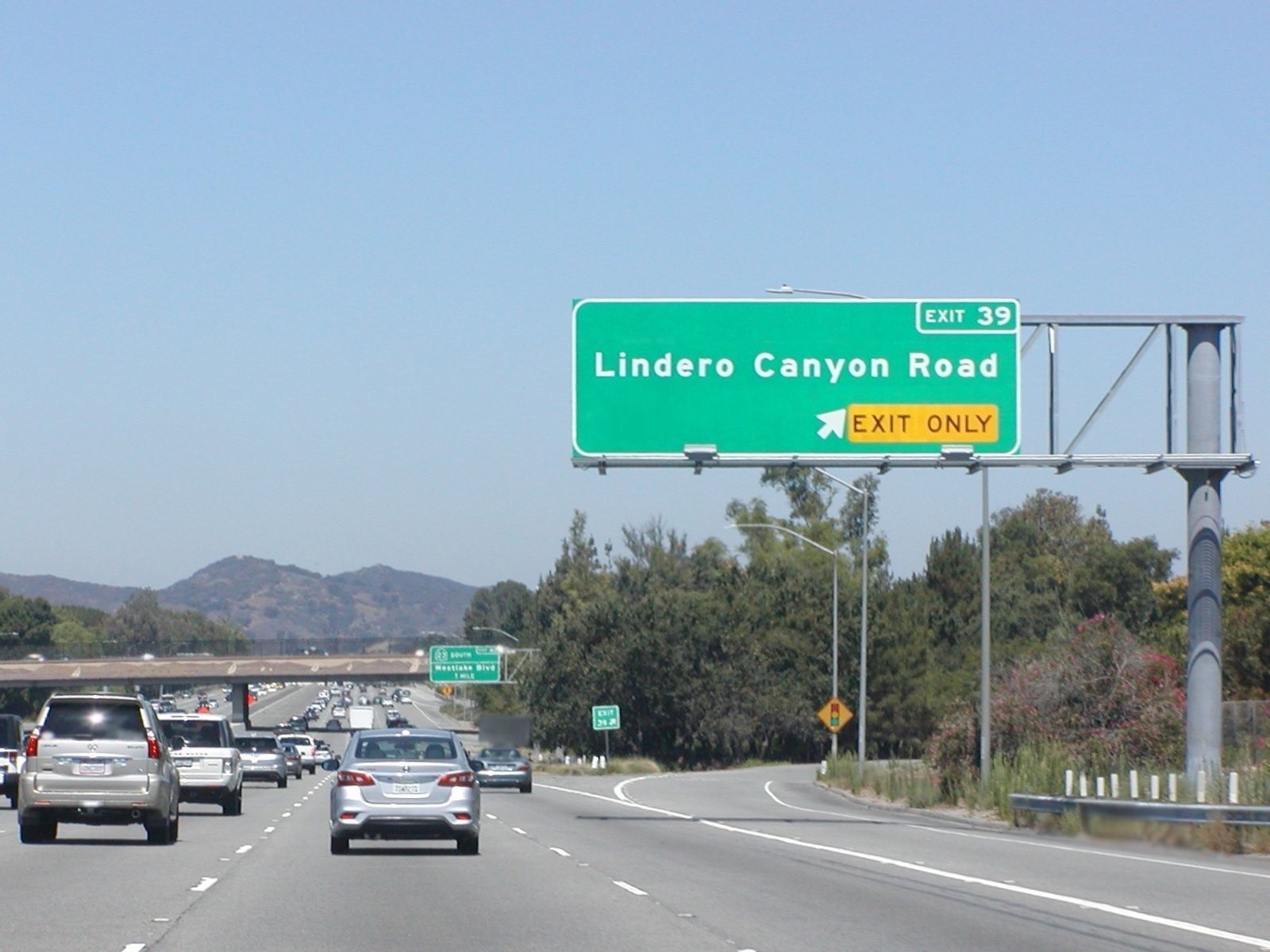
[425,749,444,758]
[366,749,386,757]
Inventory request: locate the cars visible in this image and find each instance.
[321,725,486,856]
[326,719,341,732]
[147,677,297,719]
[273,722,294,735]
[289,715,308,731]
[229,728,289,787]
[472,747,533,792]
[281,742,305,780]
[301,707,316,722]
[374,683,410,729]
[311,738,334,766]
[312,686,340,712]
[343,681,371,705]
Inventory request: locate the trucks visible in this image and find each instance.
[349,704,375,738]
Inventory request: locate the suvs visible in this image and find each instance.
[155,710,244,812]
[17,691,183,850]
[277,734,320,774]
[333,702,345,718]
[0,714,26,809]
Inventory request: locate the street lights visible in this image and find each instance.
[473,626,519,652]
[728,517,842,757]
[766,282,996,791]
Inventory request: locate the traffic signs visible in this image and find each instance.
[572,299,1022,462]
